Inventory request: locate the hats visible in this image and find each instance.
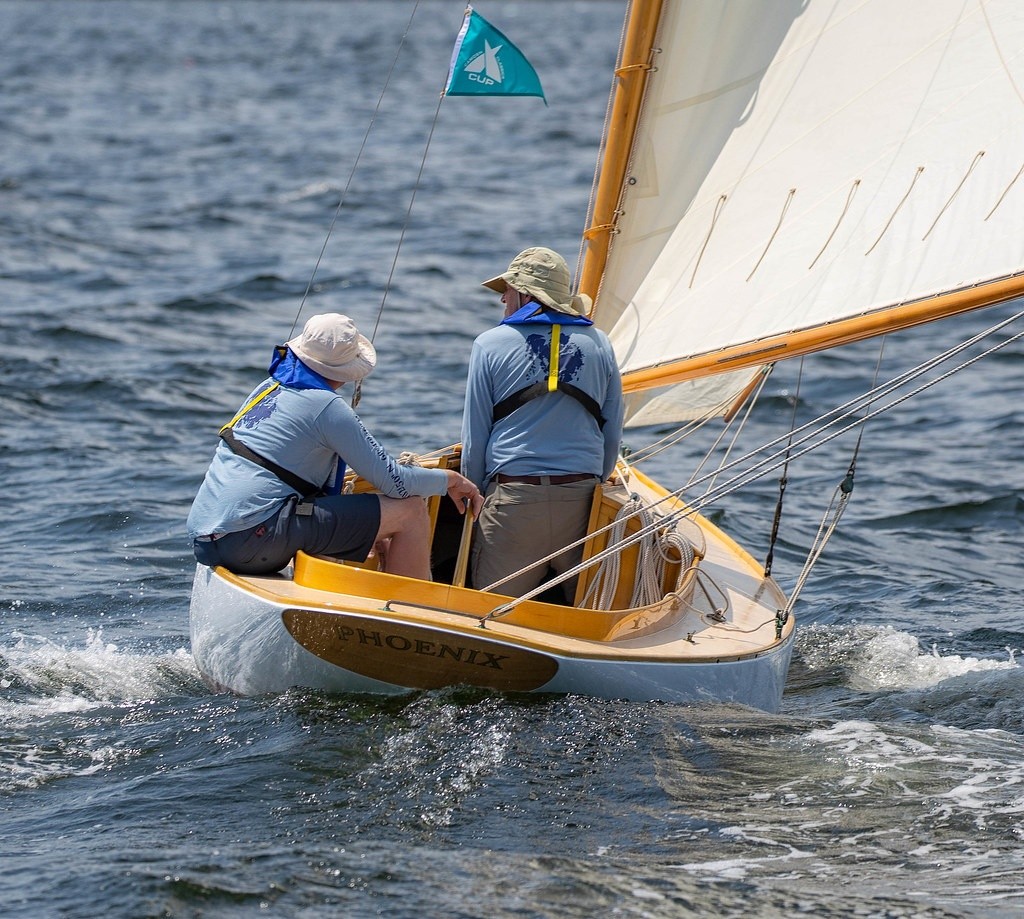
[286,314,377,382]
[482,247,579,316]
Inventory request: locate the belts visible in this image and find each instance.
[490,471,596,486]
[192,531,228,543]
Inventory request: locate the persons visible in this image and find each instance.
[461,246,623,607]
[186,312,484,582]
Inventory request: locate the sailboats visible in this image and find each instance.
[188,0,1024,714]
